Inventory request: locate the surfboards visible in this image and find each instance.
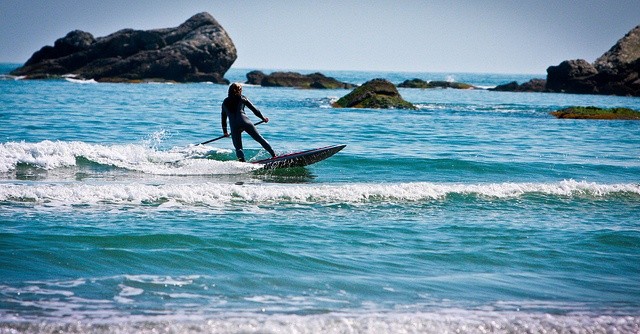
[244,144,345,170]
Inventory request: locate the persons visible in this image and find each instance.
[221,81,277,162]
